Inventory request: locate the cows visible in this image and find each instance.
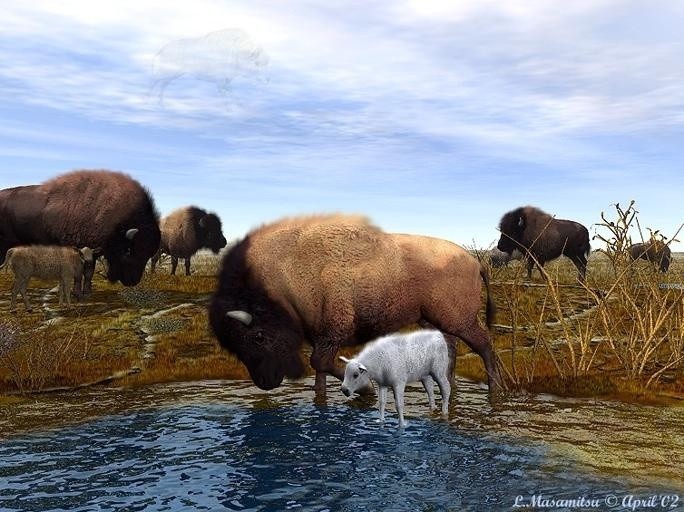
[627,240,673,275]
[207,210,497,407]
[336,327,453,427]
[0,243,102,315]
[148,203,226,277]
[0,167,161,286]
[495,204,592,284]
